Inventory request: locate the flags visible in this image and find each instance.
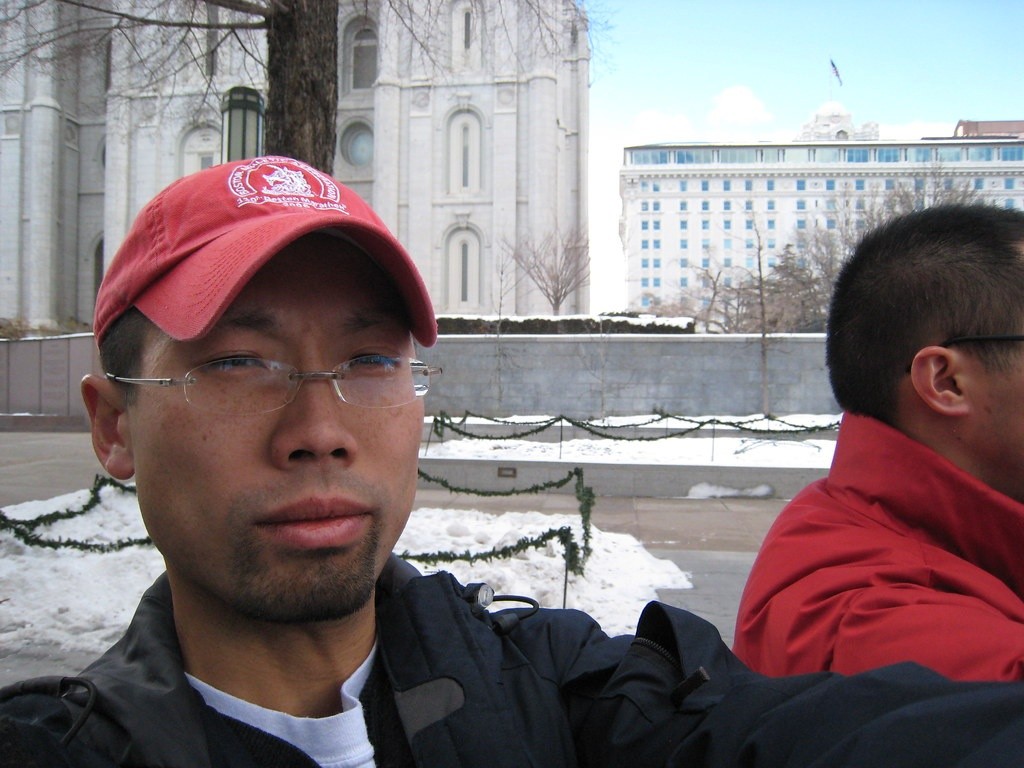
[831,60,843,85]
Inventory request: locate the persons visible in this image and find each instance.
[1,156,1024,768]
[731,205,1024,680]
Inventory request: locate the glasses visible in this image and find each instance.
[102,345,444,417]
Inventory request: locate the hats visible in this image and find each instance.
[94,155,438,347]
